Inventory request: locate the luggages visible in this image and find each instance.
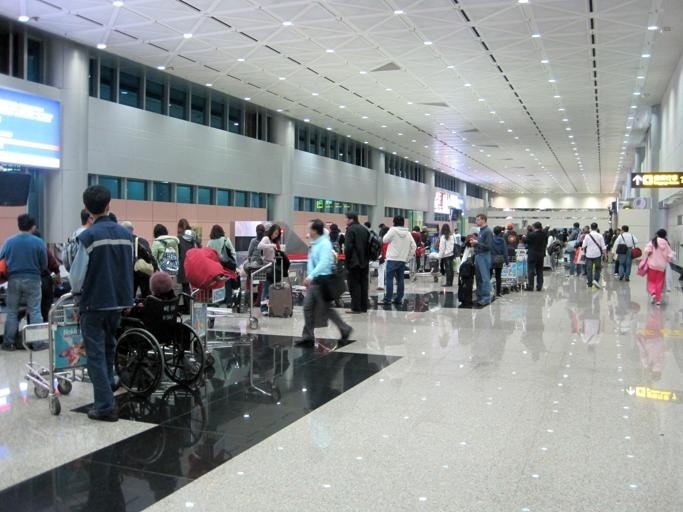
[269,256,292,317]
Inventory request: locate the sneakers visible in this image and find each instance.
[650,294,656,305]
[377,299,392,304]
[88,407,120,422]
[392,299,403,304]
[477,300,491,305]
[593,280,600,289]
[619,275,624,280]
[625,277,629,281]
[227,302,234,308]
[656,302,660,305]
[2,344,17,351]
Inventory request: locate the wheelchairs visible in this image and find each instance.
[115,292,204,399]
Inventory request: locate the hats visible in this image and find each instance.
[183,229,197,242]
[149,271,172,293]
[506,223,512,229]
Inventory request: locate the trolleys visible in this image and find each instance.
[501,252,528,295]
[205,258,273,330]
[21,290,91,417]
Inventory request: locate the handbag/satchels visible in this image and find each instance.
[565,239,575,253]
[439,259,445,275]
[492,255,504,267]
[133,259,154,277]
[454,244,461,257]
[663,263,673,291]
[219,237,236,270]
[616,245,628,255]
[573,247,586,265]
[416,246,425,257]
[636,258,648,277]
[316,274,346,302]
[601,254,604,266]
[266,262,281,283]
[631,248,642,260]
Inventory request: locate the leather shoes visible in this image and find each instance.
[293,339,314,347]
[537,288,541,291]
[524,288,533,291]
[338,327,352,343]
[346,310,361,313]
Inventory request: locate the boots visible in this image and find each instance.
[442,276,453,286]
[497,283,504,298]
[260,300,268,316]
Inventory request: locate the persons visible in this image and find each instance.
[35,230,62,322]
[304,210,639,313]
[0,214,35,350]
[637,227,676,307]
[293,218,353,346]
[64,184,291,422]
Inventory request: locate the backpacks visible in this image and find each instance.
[272,250,289,276]
[458,261,474,305]
[62,232,79,273]
[328,234,344,273]
[156,238,179,277]
[360,223,382,261]
[507,231,517,245]
